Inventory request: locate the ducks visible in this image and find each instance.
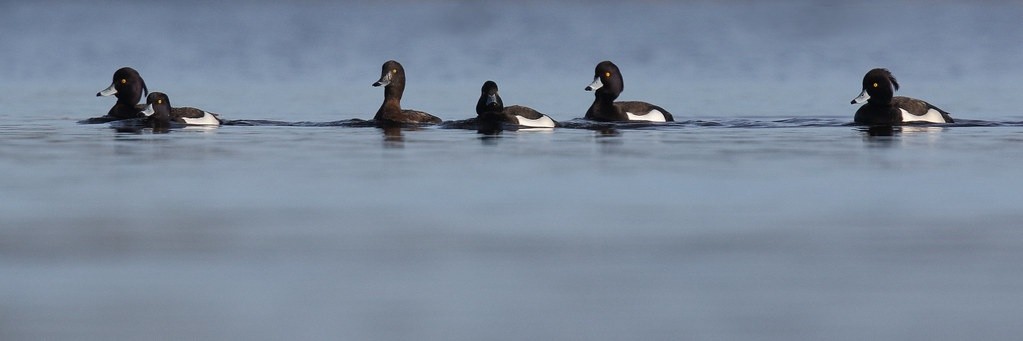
[371,60,443,123]
[96,67,149,118]
[584,60,675,123]
[475,80,560,128]
[137,92,223,125]
[851,69,954,124]
